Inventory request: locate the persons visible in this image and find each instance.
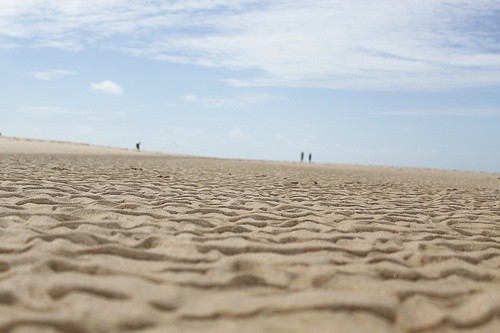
[300,152,304,161]
[309,154,311,161]
[137,144,140,149]
